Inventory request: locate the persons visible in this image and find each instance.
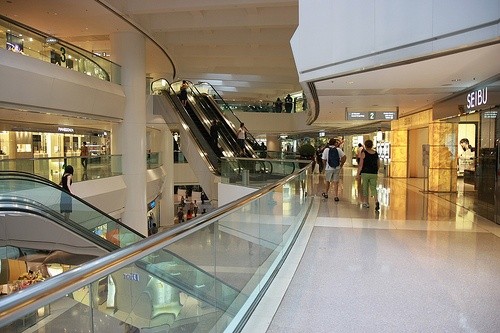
[180,80,188,106]
[285,94,292,113]
[272,102,275,113]
[460,138,475,152]
[316,139,346,201]
[237,123,246,155]
[249,240,254,256]
[357,140,380,211]
[177,188,207,223]
[210,120,218,148]
[275,97,283,113]
[80,142,88,170]
[59,165,74,219]
[356,143,364,175]
[151,223,158,234]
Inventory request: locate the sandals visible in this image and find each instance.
[321,192,329,198]
[334,197,339,201]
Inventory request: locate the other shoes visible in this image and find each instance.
[375,201,379,211]
[362,202,370,208]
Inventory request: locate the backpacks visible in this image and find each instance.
[326,146,340,167]
